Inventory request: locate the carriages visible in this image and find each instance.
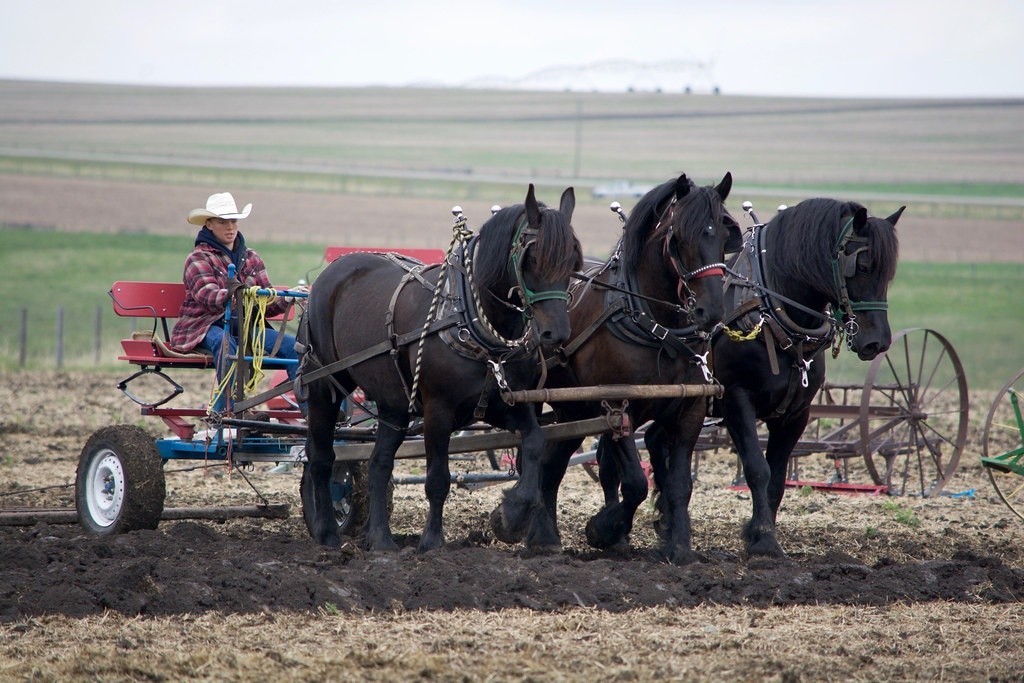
[74,172,909,559]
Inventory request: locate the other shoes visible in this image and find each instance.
[240,410,269,437]
[304,410,345,422]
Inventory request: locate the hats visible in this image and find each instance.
[187,192,252,226]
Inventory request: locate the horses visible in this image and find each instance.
[488,163,746,568]
[295,181,588,562]
[594,195,910,572]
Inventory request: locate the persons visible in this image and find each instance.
[172,191,346,437]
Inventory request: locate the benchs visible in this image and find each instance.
[107,281,303,407]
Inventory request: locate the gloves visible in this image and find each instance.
[284,288,309,304]
